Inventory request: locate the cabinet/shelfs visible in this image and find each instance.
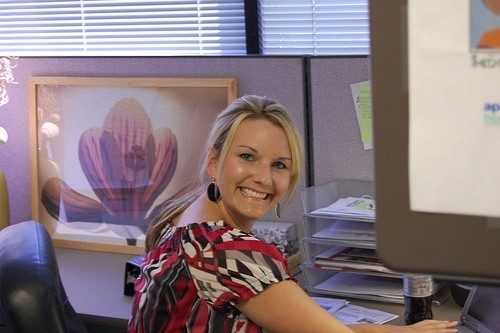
[299,178,446,304]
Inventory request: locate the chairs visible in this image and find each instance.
[1,220,89,333]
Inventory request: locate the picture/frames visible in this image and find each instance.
[27,75,238,256]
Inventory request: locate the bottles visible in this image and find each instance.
[402,273,434,326]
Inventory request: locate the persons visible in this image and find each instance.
[126,92,462,333]
